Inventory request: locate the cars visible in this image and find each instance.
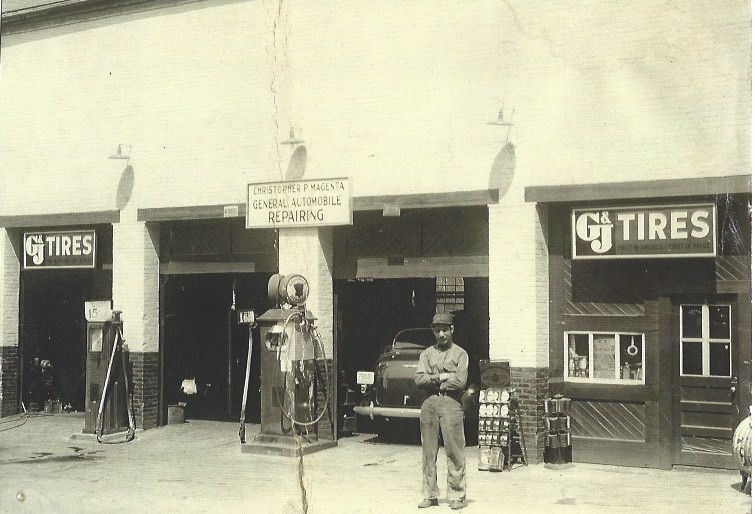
[351,323,485,445]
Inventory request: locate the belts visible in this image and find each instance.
[437,390,447,396]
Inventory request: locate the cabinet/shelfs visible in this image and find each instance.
[475,387,528,472]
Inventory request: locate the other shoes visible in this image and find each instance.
[418,498,438,508]
[451,500,464,509]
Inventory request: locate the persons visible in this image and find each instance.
[413,314,469,512]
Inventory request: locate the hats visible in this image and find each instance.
[432,314,453,325]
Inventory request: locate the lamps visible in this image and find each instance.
[488,104,517,126]
[277,126,307,147]
[108,143,131,161]
